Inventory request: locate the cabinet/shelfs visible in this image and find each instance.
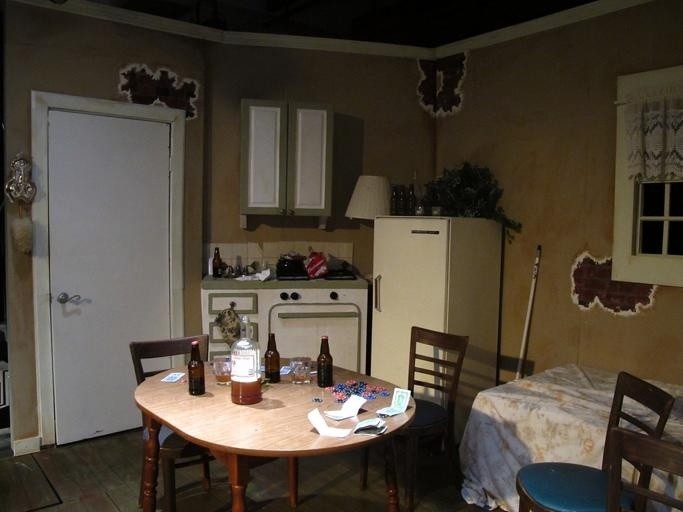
[241,97,334,216]
[200,289,265,360]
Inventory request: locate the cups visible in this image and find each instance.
[414,203,425,215]
[289,357,311,384]
[433,206,442,215]
[213,353,233,385]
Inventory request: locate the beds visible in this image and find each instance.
[457,365,683,512]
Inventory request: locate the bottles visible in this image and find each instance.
[264,332,281,383]
[188,340,205,395]
[407,183,416,215]
[390,183,397,215]
[212,247,223,278]
[230,316,262,406]
[397,184,406,215]
[318,336,333,388]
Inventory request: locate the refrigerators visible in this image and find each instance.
[372,216,503,447]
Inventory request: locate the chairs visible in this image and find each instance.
[130,333,209,512]
[391,326,471,512]
[608,426,683,512]
[515,370,674,512]
